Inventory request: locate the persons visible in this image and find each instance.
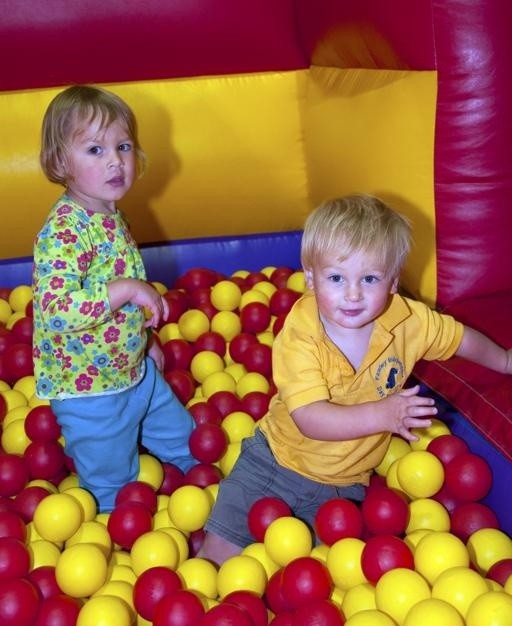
[198,193,512,566]
[32,85,200,515]
